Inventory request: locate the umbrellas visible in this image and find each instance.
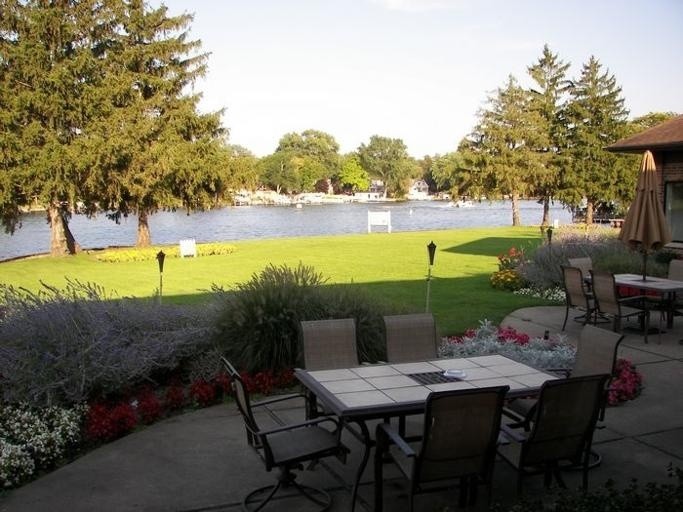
[617,149,673,327]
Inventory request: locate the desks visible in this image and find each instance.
[288,351,568,511]
[585,271,682,345]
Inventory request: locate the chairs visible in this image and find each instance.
[589,268,651,345]
[558,265,599,330]
[381,312,442,451]
[567,256,610,321]
[212,355,350,511]
[376,385,510,511]
[655,259,683,320]
[297,317,364,436]
[524,323,624,470]
[499,372,613,496]
